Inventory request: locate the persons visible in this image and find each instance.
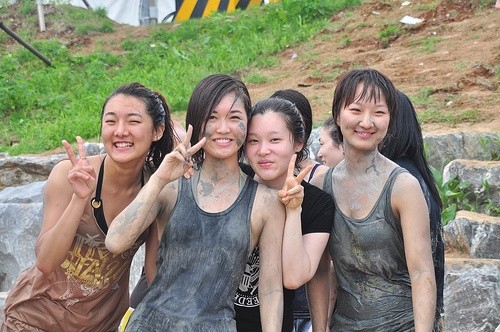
[0,82,177,332]
[106,74,284,332]
[377,91,445,332]
[308,68,436,332]
[232,89,345,332]
